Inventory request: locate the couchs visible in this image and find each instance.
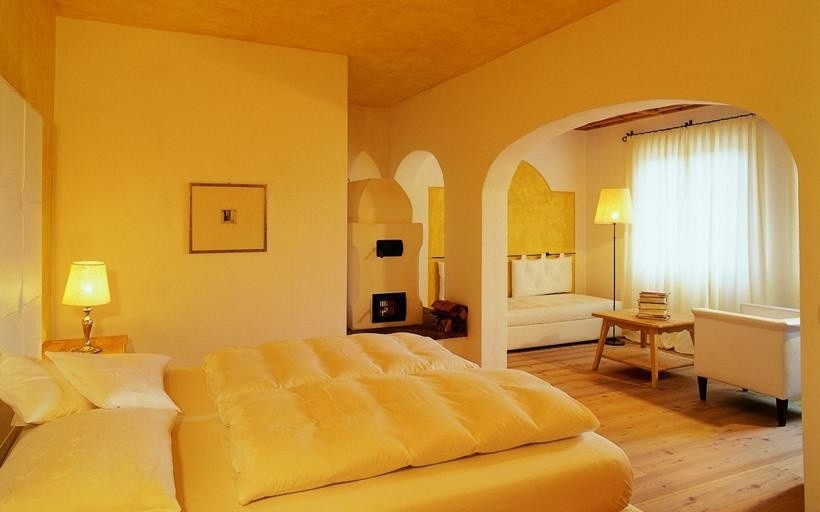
[506,293,622,351]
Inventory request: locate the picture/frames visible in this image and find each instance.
[188,183,268,253]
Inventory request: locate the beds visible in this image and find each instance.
[0,331,634,512]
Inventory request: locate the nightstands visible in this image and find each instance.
[42,334,128,352]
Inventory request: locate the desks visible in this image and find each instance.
[592,309,694,388]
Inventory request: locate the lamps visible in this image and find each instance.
[593,187,634,346]
[61,260,111,353]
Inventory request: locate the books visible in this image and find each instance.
[636,289,672,321]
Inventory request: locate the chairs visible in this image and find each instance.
[691,303,801,427]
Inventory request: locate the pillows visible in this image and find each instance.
[0,408,182,511]
[44,351,180,407]
[0,352,94,424]
